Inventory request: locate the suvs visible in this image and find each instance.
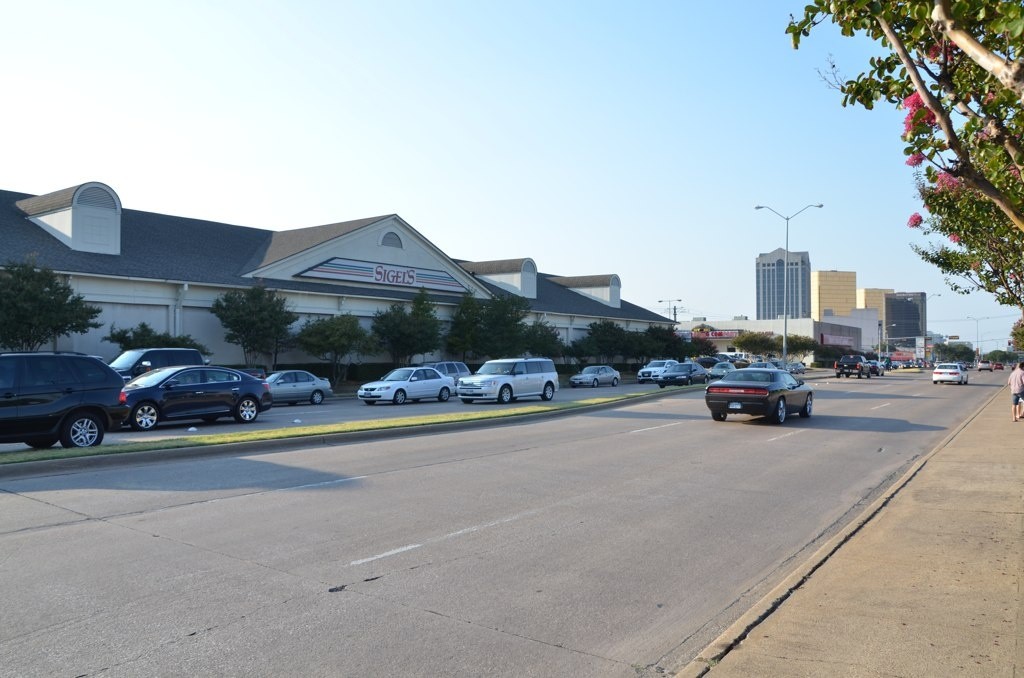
[420,361,473,383]
[456,357,560,406]
[107,348,205,386]
[0,351,130,451]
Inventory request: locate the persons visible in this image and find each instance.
[1008,362,1024,421]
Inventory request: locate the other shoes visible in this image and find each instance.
[1020,416,1024,418]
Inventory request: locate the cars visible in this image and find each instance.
[977,359,993,372]
[1012,363,1017,370]
[713,352,781,369]
[694,357,722,369]
[993,362,1005,370]
[121,364,272,431]
[834,354,872,379]
[262,369,334,405]
[658,361,711,389]
[881,358,940,370]
[636,359,680,384]
[708,362,737,379]
[750,362,778,372]
[569,365,622,388]
[703,368,815,424]
[867,360,886,376]
[933,363,970,385]
[356,365,457,405]
[786,362,806,375]
[956,361,973,371]
[236,368,269,381]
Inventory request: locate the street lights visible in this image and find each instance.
[878,324,897,361]
[658,299,683,320]
[753,202,826,364]
[907,294,942,363]
[966,316,990,362]
